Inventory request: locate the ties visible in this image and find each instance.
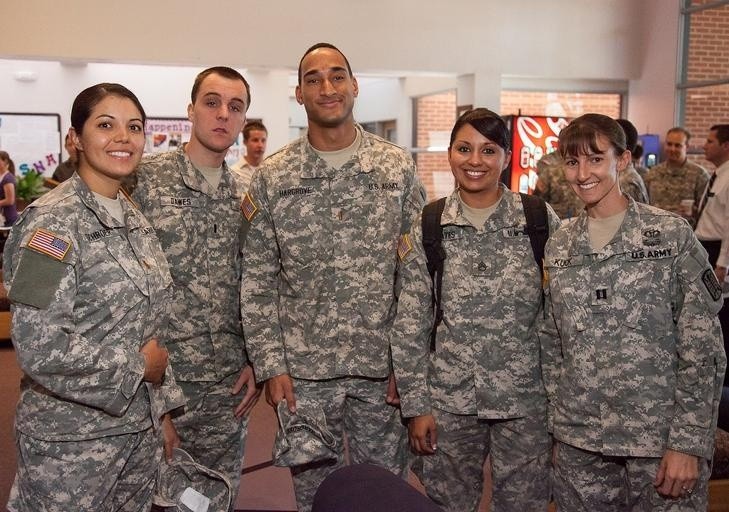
[692,171,716,231]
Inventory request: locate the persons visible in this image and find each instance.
[612,118,650,208]
[0,81,189,511]
[0,150,19,239]
[233,40,429,512]
[230,121,270,204]
[532,131,585,224]
[536,112,725,509]
[689,120,728,390]
[108,63,265,511]
[643,127,713,234]
[386,106,569,511]
[50,134,79,183]
[630,143,649,175]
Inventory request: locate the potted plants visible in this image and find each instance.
[15,170,46,211]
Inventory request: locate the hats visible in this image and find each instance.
[273,396,338,467]
[152,447,235,512]
